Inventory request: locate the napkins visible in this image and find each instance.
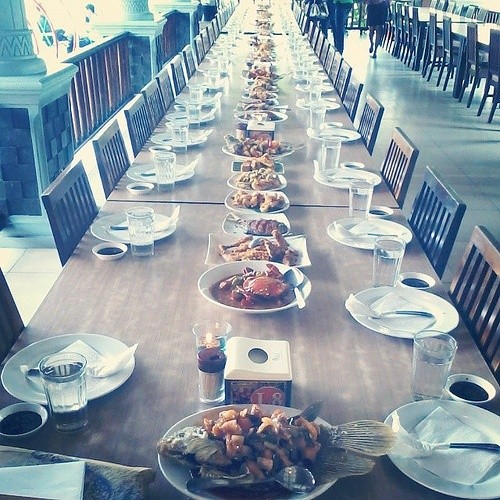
[57,335,139,378]
[174,156,199,177]
[203,107,218,119]
[332,218,390,241]
[191,129,214,141]
[371,290,437,336]
[294,83,308,92]
[403,407,500,487]
[0,458,91,500]
[296,96,308,108]
[109,206,180,240]
[206,91,222,102]
[313,160,348,182]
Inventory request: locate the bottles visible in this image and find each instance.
[196,347,227,405]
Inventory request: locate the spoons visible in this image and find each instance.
[284,267,306,310]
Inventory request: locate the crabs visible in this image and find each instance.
[233,271,290,302]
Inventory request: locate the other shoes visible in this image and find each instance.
[369,43,373,53]
[372,54,377,58]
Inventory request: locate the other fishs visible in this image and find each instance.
[226,212,288,236]
[242,68,293,81]
[224,133,307,157]
[155,399,398,483]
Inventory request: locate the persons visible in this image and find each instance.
[197,0,218,22]
[84,4,104,43]
[303,0,353,55]
[366,0,392,58]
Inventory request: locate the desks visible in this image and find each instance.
[0,12,500,500]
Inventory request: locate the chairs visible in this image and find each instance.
[0,0,500,400]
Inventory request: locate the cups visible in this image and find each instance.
[372,236,406,288]
[349,181,374,218]
[125,205,155,261]
[320,136,342,181]
[153,152,176,194]
[193,319,232,351]
[410,329,458,402]
[38,351,89,433]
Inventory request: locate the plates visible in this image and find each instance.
[150,0,361,160]
[367,205,395,220]
[126,182,155,195]
[198,160,311,314]
[90,213,177,244]
[158,403,339,500]
[327,217,412,250]
[91,242,128,261]
[349,287,460,339]
[0,402,48,439]
[1,333,135,406]
[383,400,500,500]
[398,272,436,291]
[444,373,496,406]
[312,169,382,190]
[127,164,194,185]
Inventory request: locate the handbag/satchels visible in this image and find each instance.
[309,0,329,20]
